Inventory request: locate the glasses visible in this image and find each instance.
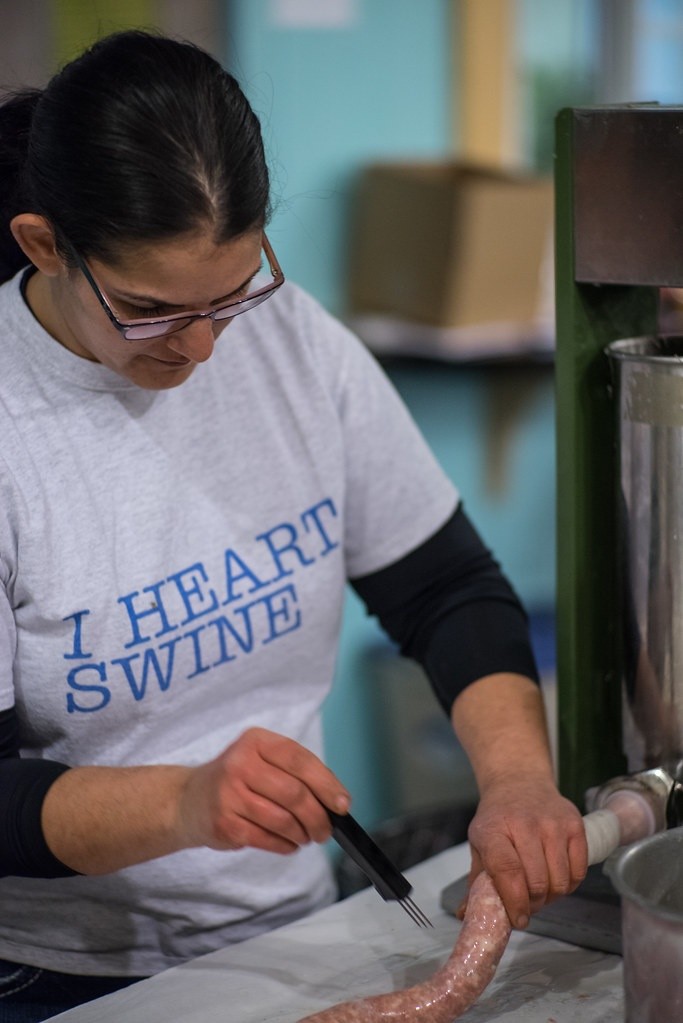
[55,227,286,341]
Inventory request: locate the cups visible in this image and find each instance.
[613,824,683,1023]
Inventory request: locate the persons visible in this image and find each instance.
[0,30,589,1022]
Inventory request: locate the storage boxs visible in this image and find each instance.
[348,163,554,329]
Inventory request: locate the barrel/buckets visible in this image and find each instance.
[603,332,682,778]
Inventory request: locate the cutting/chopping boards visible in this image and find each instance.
[440,860,622,955]
